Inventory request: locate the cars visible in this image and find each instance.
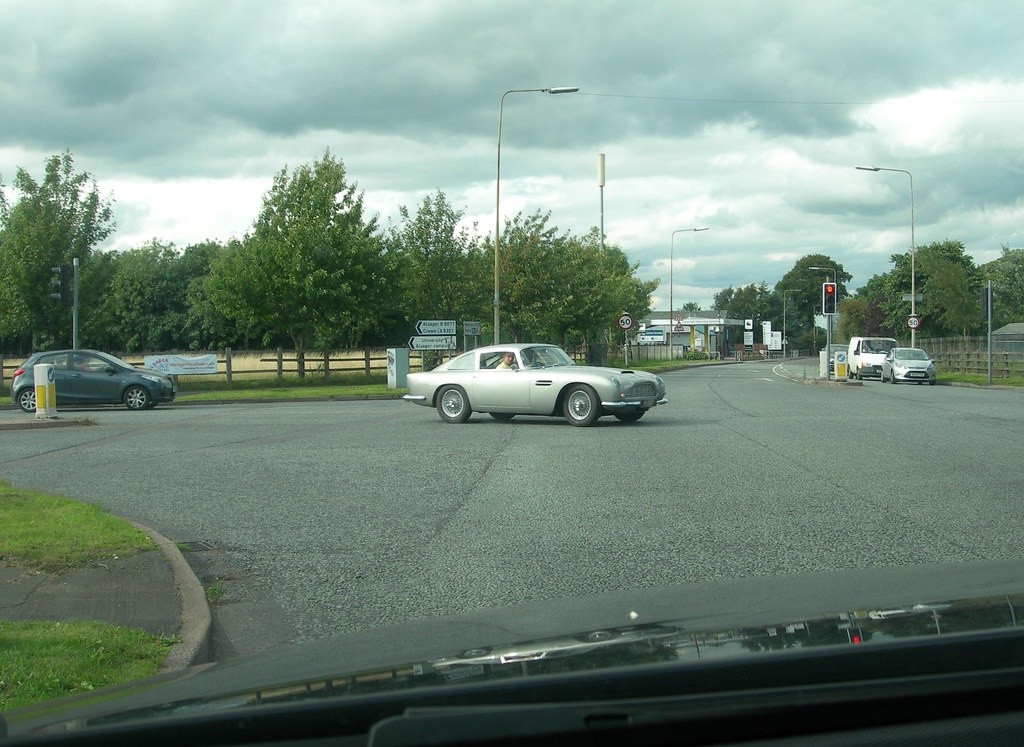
[730,349,736,355]
[880,347,936,385]
[402,343,670,427]
[11,351,177,412]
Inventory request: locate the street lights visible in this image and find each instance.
[494,86,581,345]
[670,227,709,361]
[784,290,802,357]
[855,166,916,348]
[808,267,837,345]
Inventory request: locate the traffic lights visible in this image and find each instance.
[823,283,838,316]
[50,264,69,304]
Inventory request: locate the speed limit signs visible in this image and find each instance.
[618,315,632,330]
[907,317,919,328]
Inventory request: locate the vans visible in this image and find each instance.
[821,345,848,371]
[847,336,900,381]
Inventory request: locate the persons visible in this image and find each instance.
[863,341,874,351]
[496,352,515,369]
[74,354,97,371]
